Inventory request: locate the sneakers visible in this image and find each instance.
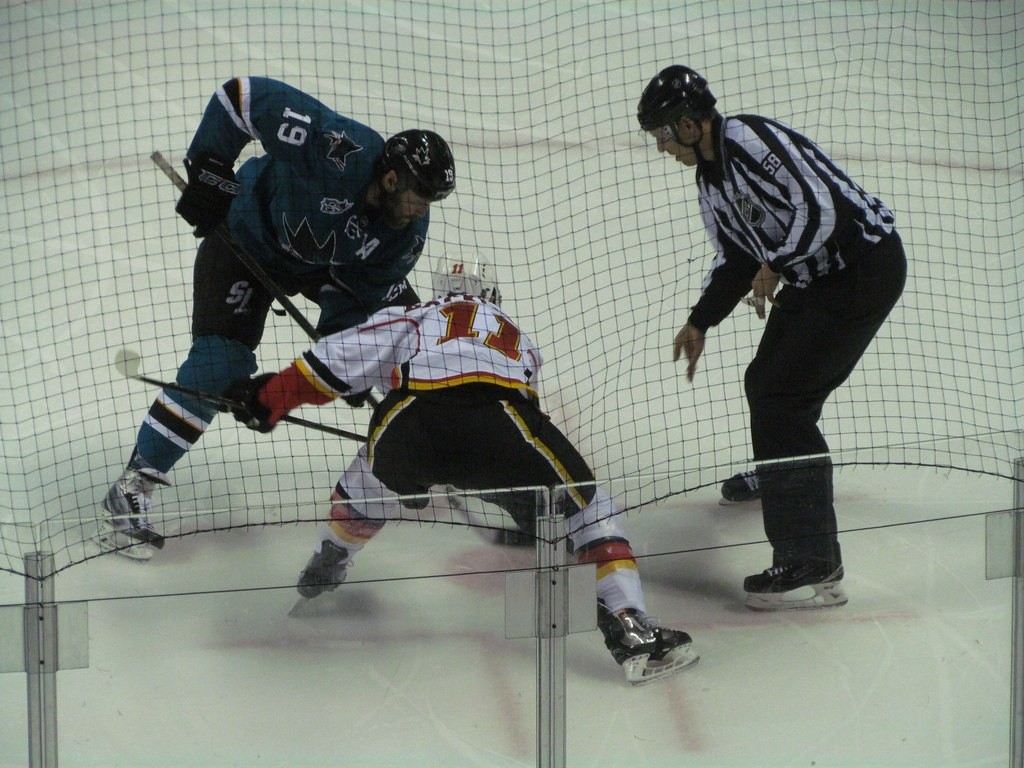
[88,445,170,561]
[593,595,699,684]
[288,540,353,618]
[717,469,763,505]
[742,553,847,611]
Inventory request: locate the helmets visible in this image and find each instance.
[379,129,455,203]
[430,246,503,308]
[637,64,708,130]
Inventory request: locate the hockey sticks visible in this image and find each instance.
[148,147,536,548]
[114,346,370,446]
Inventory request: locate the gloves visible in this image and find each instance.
[225,373,281,433]
[176,158,240,237]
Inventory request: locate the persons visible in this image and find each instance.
[234,247,700,688]
[89,77,456,562]
[637,64,909,613]
